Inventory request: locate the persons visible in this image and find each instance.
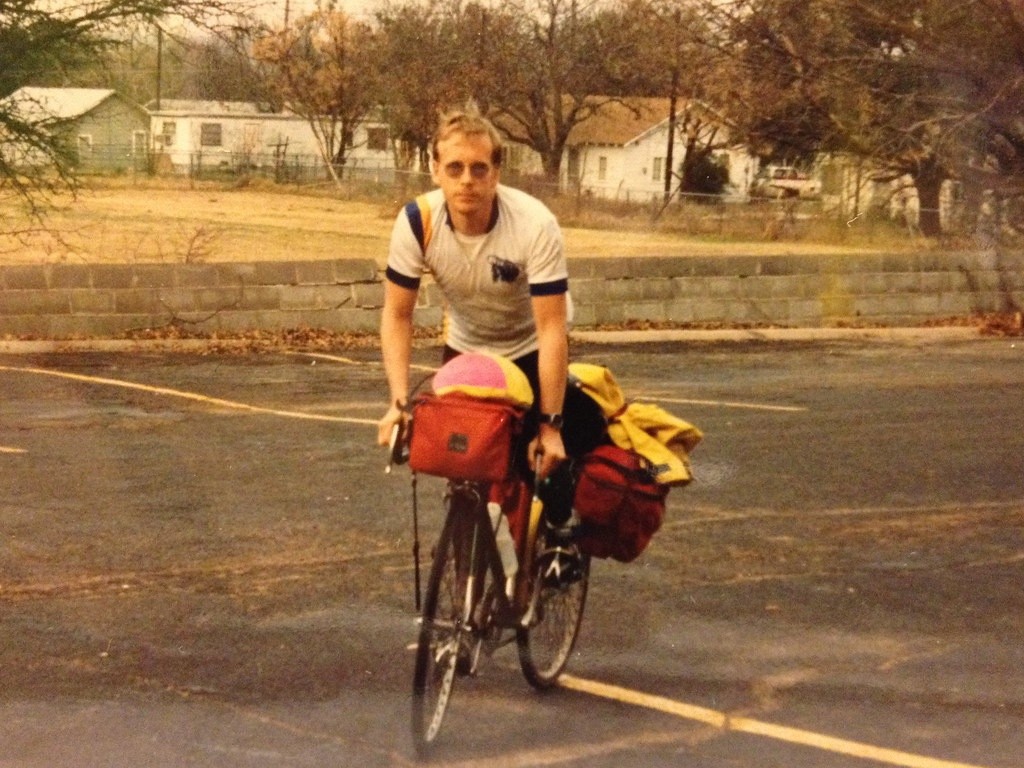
[375,112,580,676]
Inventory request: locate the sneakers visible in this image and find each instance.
[540,526,576,586]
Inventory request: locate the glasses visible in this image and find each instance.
[438,161,497,179]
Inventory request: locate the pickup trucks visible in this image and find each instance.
[757,165,820,200]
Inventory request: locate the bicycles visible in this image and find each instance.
[380,388,593,760]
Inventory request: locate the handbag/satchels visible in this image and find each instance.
[408,395,522,484]
[577,446,668,565]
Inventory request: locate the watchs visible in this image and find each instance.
[538,413,565,430]
